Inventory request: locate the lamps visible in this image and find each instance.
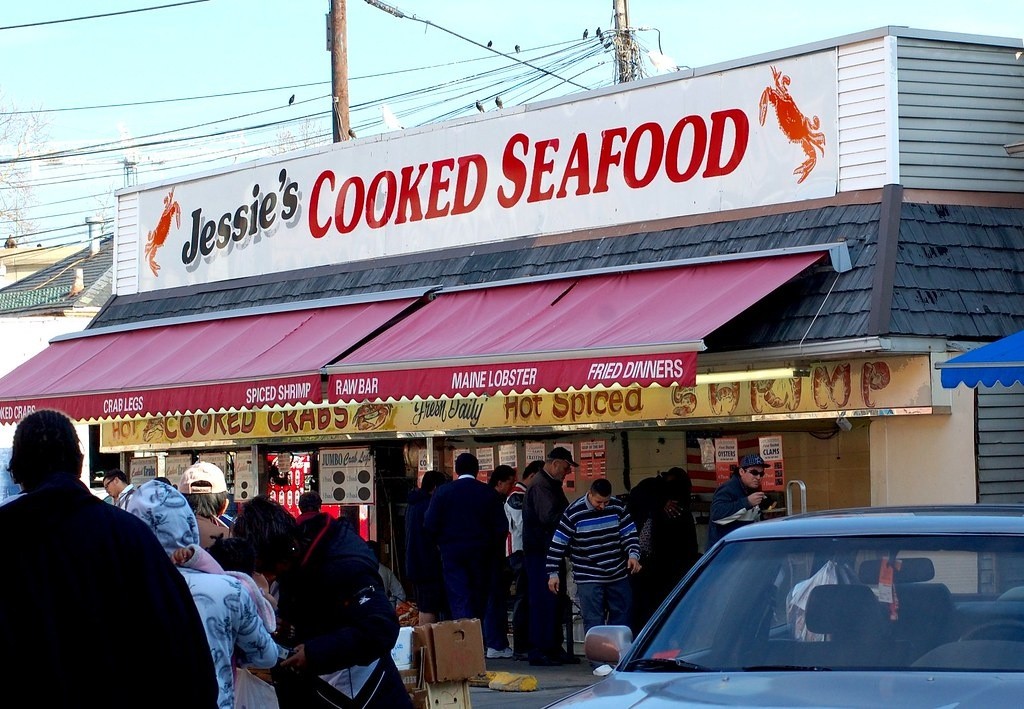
[79,359,815,426]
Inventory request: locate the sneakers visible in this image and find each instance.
[487,647,513,658]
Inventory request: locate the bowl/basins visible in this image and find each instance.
[762,507,787,519]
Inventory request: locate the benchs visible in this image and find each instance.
[868,583,1024,660]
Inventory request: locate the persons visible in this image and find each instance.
[0,409,219,709]
[402,447,772,668]
[102,460,415,709]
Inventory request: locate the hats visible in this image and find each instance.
[661,467,686,480]
[179,461,228,494]
[548,447,579,467]
[739,454,771,468]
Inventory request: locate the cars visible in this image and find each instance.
[538,500,1024,709]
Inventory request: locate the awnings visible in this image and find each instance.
[0,297,420,425]
[934,330,1024,388]
[325,251,826,405]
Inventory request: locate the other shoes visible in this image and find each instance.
[530,657,554,666]
[555,652,580,664]
[514,653,528,660]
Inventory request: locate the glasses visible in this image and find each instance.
[104,478,115,488]
[745,469,764,477]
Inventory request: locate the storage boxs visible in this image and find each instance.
[392,617,486,709]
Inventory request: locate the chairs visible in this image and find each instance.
[772,584,919,665]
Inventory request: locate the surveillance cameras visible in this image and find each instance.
[836,417,852,431]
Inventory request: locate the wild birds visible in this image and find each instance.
[476,99,486,113]
[348,127,356,140]
[495,96,503,109]
[515,45,521,54]
[289,94,295,106]
[583,27,604,44]
[377,103,404,132]
[487,40,493,48]
